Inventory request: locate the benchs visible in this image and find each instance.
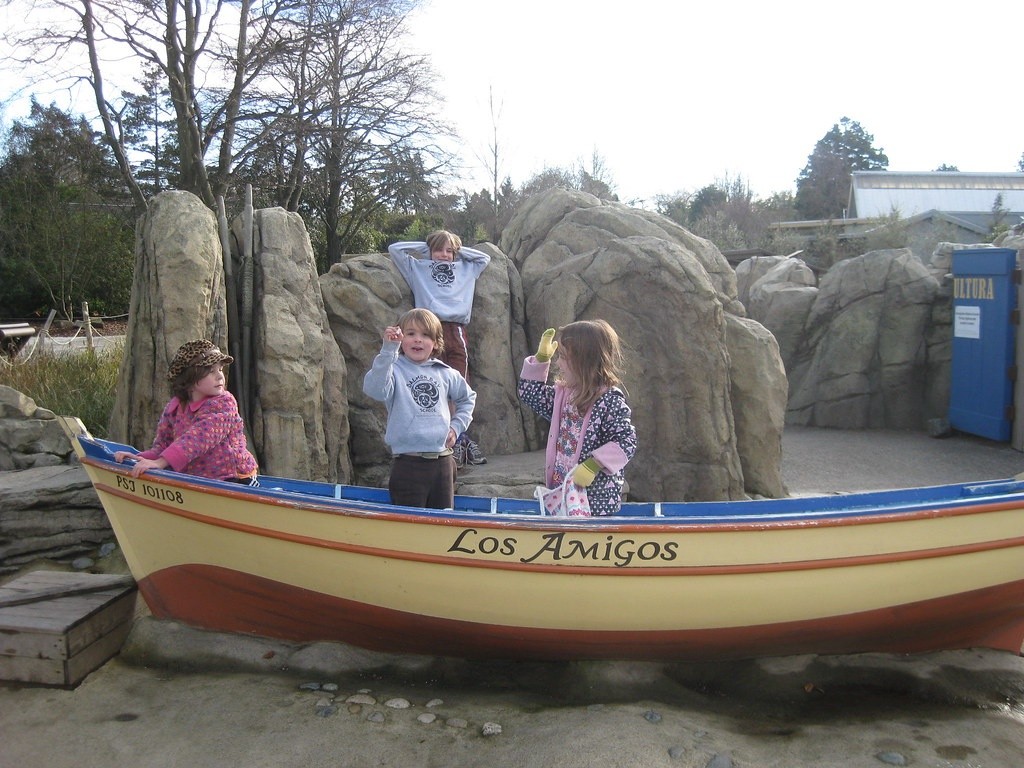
[768,217,888,237]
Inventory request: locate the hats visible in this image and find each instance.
[166,340,234,382]
[533,467,591,517]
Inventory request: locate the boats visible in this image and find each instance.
[46,410,1023,660]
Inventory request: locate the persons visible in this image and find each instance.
[389,230,491,468]
[518,320,638,517]
[115,338,259,488]
[363,309,477,509]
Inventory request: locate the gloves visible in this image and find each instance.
[574,457,602,487]
[535,328,559,362]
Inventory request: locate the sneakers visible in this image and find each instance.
[466,441,487,466]
[452,442,466,469]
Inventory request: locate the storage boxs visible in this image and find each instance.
[0,570,138,689]
